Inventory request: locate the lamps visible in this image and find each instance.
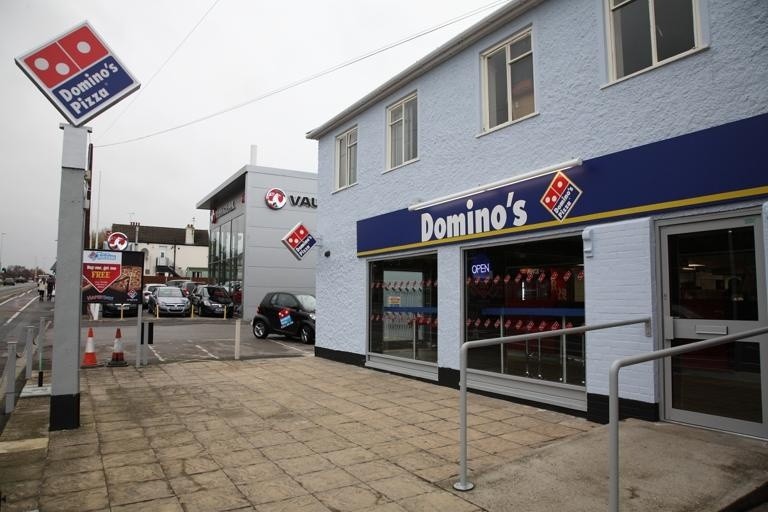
[406,156,583,212]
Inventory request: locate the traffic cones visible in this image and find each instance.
[106,327,130,369]
[79,327,103,369]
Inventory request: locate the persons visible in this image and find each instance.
[46,275,55,301]
[38,278,48,302]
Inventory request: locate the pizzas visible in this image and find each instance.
[109,266,142,292]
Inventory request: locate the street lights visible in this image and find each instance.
[0,232,6,266]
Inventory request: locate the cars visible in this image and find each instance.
[249,289,315,345]
[2,278,15,286]
[100,303,138,319]
[213,280,242,293]
[142,282,168,305]
[669,305,702,321]
[146,286,191,316]
[16,275,27,284]
[163,279,235,317]
